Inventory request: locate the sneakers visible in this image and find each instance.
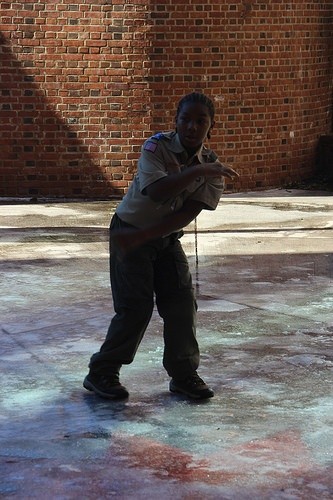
[170,369,215,400]
[82,370,129,397]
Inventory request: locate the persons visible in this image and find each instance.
[80,93,240,400]
